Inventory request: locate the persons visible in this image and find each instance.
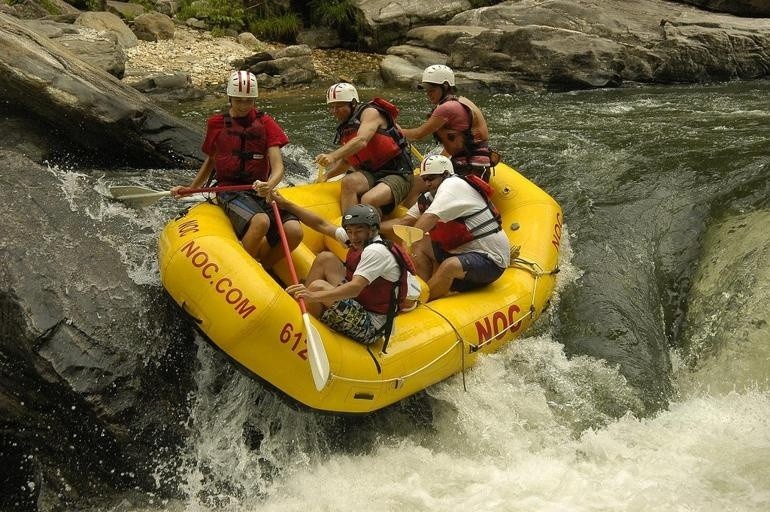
[170,70,303,271]
[316,83,414,216]
[266,187,407,345]
[378,154,510,300]
[402,64,491,209]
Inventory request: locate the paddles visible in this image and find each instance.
[110,185,257,207]
[272,201,330,392]
[392,225,423,257]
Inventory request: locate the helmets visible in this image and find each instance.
[420,155,454,176]
[326,83,360,105]
[226,70,258,98]
[422,64,455,87]
[342,204,381,230]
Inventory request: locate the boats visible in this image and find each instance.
[159,160,563,419]
